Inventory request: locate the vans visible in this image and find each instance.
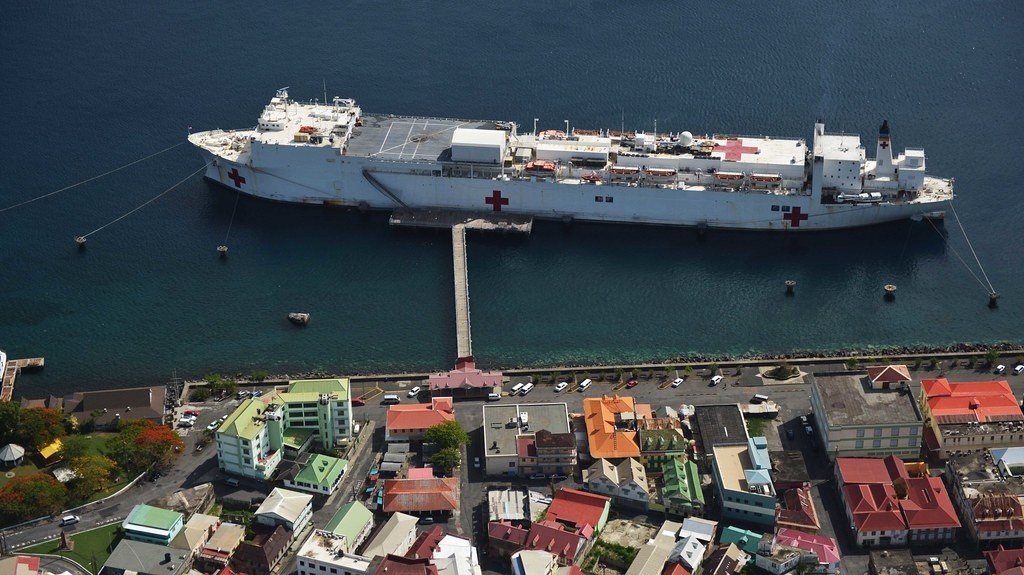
[1014,365,1024,374]
[995,365,1005,374]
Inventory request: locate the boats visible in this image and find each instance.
[185,74,959,231]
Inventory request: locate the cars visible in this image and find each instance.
[175,374,724,434]
